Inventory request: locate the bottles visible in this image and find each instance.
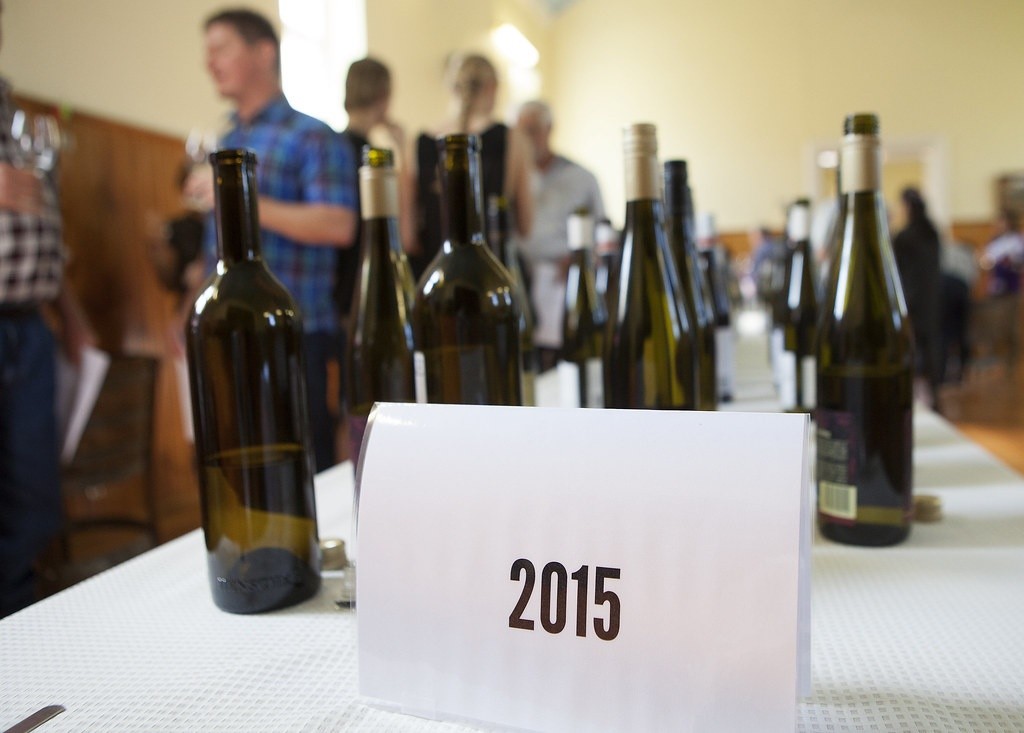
[187,114,918,618]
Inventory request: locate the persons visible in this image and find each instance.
[0,73,72,623]
[889,186,944,417]
[330,58,394,423]
[695,213,733,269]
[387,55,540,405]
[751,226,785,280]
[179,5,360,480]
[515,98,609,373]
[931,239,979,378]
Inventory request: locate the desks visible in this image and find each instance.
[0,309,1024,733]
